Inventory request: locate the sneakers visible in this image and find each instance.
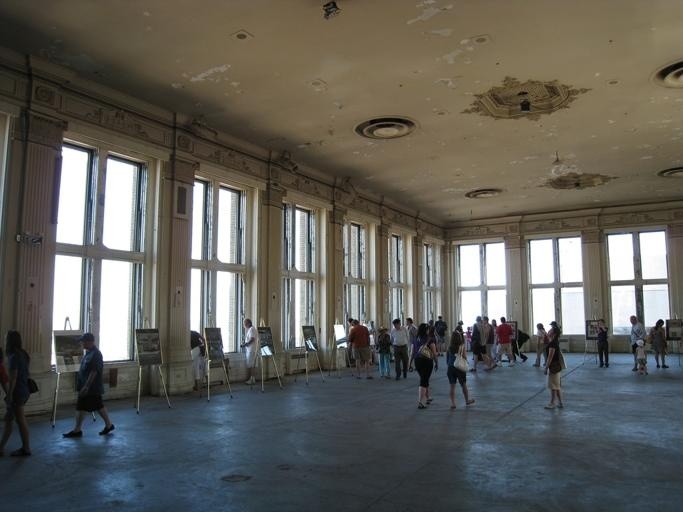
[469,357,528,373]
[245,378,256,385]
[451,406,456,409]
[556,402,564,408]
[532,363,540,367]
[657,365,660,368]
[466,399,475,405]
[418,402,427,409]
[632,367,638,372]
[367,374,408,380]
[437,353,444,357]
[544,403,555,410]
[427,398,433,404]
[599,364,609,368]
[662,365,669,368]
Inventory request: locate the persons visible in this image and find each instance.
[650,319,670,369]
[595,318,611,369]
[189,330,208,391]
[0,329,34,459]
[239,318,259,385]
[0,346,10,397]
[629,315,648,371]
[347,309,568,411]
[61,332,117,438]
[634,339,649,376]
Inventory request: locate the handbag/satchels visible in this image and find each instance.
[453,353,468,372]
[559,352,568,370]
[419,345,434,360]
[26,377,39,394]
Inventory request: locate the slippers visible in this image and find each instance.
[11,448,32,456]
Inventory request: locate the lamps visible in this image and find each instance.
[647,55,683,182]
[352,113,416,141]
[463,187,501,201]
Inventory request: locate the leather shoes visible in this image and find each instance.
[99,424,115,435]
[63,430,83,438]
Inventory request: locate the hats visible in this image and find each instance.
[378,326,388,333]
[79,333,95,341]
[636,340,644,347]
